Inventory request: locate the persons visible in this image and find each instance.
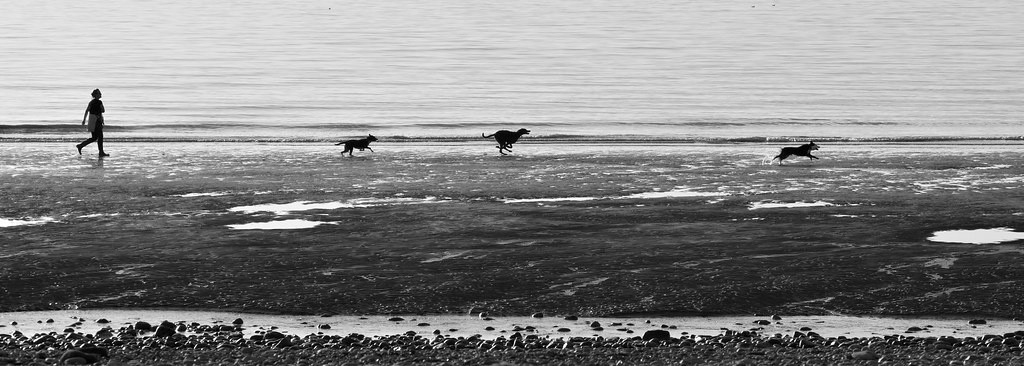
[76,89,110,157]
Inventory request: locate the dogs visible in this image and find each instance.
[772,140,820,165]
[482,128,530,156]
[335,134,378,158]
